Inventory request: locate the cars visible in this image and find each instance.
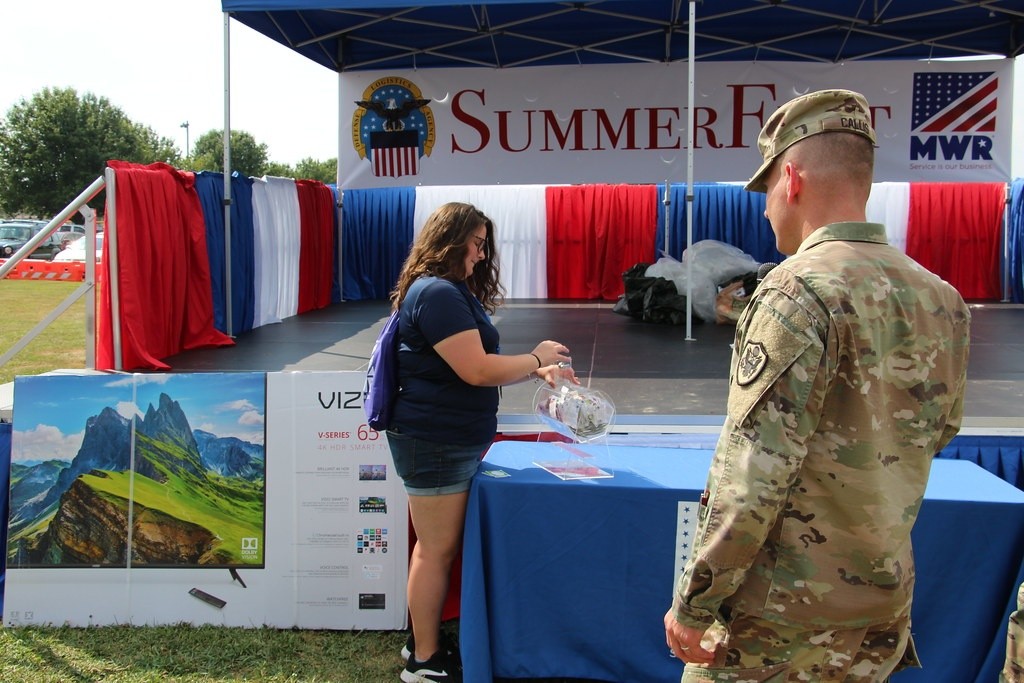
[1,219,104,262]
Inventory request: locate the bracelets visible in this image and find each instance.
[531,354,541,368]
[528,374,531,380]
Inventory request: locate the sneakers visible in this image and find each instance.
[399,648,463,683]
[401,627,463,669]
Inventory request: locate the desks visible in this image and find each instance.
[457,440,1024,683]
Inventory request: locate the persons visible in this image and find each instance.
[665,89,971,683]
[386,202,580,683]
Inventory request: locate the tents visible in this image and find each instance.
[221,0,1024,337]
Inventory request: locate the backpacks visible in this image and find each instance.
[363,309,399,431]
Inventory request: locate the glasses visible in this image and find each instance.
[473,235,486,252]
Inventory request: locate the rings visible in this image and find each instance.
[681,646,689,650]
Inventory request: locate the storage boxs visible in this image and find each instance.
[3,370,411,630]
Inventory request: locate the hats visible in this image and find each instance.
[743,89,880,193]
